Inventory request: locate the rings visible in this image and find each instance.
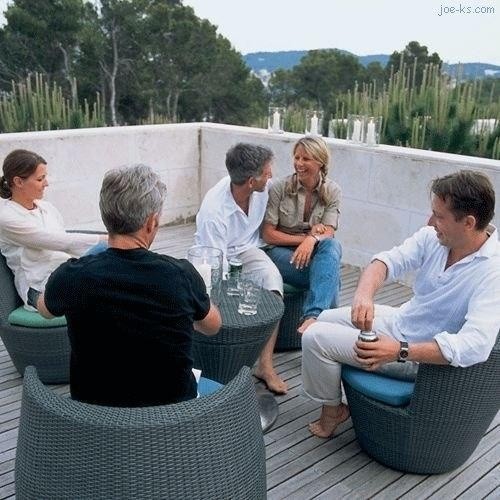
[317,228,319,232]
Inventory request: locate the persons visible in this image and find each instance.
[261,134,344,337]
[35,162,223,407]
[0,148,107,313]
[194,141,291,395]
[298,168,499,439]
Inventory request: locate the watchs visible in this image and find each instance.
[397,341,409,363]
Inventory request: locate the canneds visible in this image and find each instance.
[357,330,378,368]
[229,258,242,280]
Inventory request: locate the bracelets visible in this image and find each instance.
[312,234,321,242]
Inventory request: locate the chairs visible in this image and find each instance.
[13,361,268,500]
[341,331,500,475]
[1,252,80,384]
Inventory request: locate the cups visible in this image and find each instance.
[227,256,243,296]
[237,273,263,315]
[188,246,223,308]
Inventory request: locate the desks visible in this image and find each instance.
[182,273,286,435]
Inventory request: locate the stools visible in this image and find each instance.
[229,266,340,353]
